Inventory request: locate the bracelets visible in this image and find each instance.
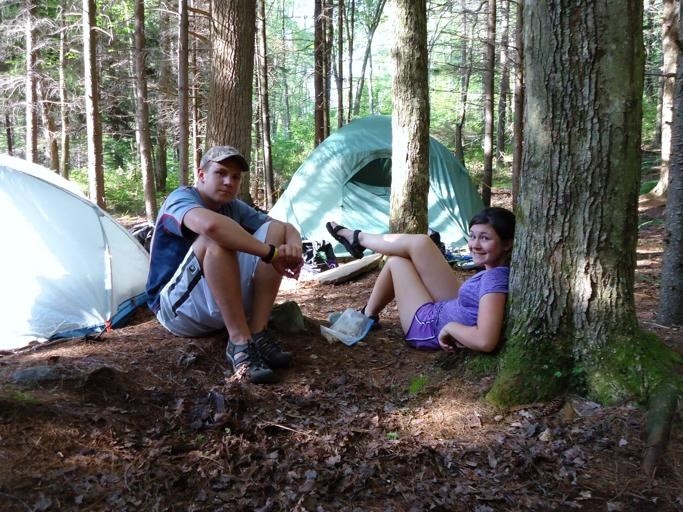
[270,247,278,263]
[262,244,275,264]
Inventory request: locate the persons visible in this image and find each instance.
[143,146,308,383]
[328,207,512,353]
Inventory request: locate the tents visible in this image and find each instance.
[260,114,488,270]
[0,152,157,360]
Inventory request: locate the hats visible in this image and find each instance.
[199,145,249,174]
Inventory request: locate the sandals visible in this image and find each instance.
[354,306,379,326]
[325,221,365,260]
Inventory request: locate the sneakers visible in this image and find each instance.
[251,330,293,367]
[225,340,273,384]
